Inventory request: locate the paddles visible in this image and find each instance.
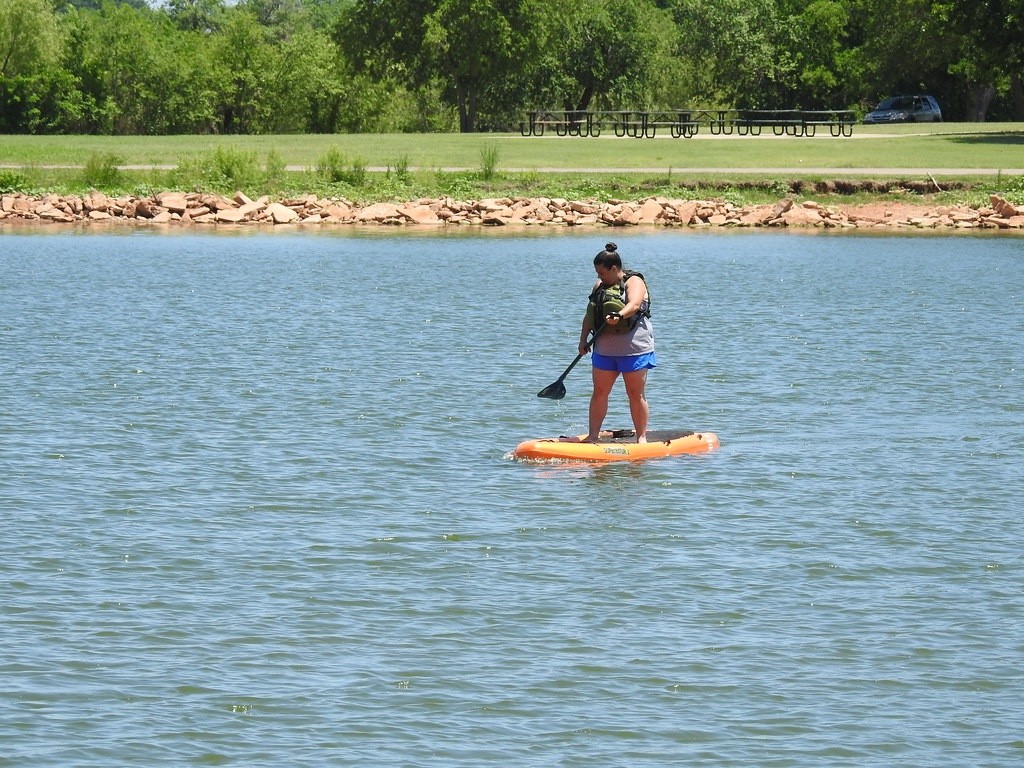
[536,311,622,400]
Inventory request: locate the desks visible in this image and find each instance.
[518,110,857,136]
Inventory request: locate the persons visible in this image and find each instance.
[578,242,656,443]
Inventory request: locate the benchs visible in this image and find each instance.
[519,120,859,136]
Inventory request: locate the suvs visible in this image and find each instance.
[863,94,941,124]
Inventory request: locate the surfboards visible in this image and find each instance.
[513,427,722,463]
